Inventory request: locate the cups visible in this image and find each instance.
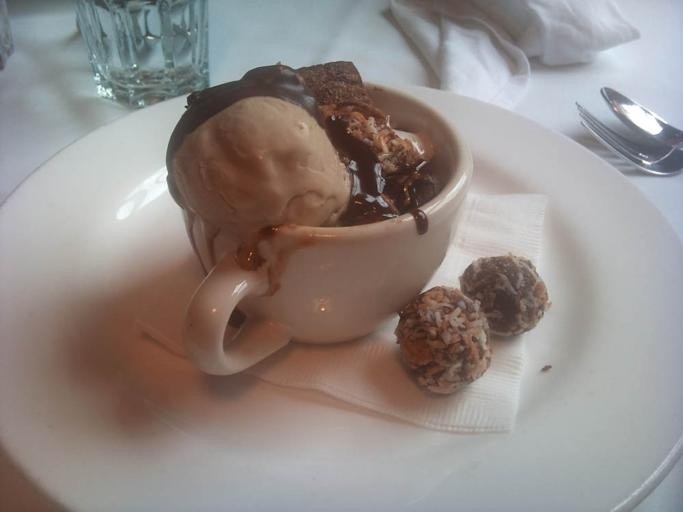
[180,79,467,376]
[73,2,211,110]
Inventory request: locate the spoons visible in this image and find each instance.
[599,83,683,149]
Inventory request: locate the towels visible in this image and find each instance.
[386,1,647,123]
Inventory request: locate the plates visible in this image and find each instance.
[0,71,682,512]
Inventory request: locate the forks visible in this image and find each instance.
[575,101,683,179]
